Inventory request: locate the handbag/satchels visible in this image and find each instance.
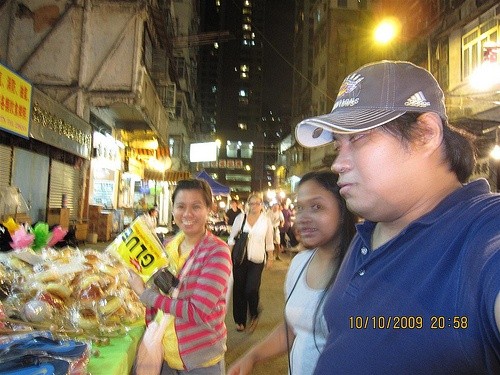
[132,320,163,375]
[231,213,249,266]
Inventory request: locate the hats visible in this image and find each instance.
[295,60,447,149]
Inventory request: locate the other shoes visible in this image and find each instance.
[236,323,246,331]
[276,257,280,260]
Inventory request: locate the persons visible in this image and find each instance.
[128,178,233,374]
[146,191,301,261]
[226,171,358,375]
[227,194,274,332]
[295,60,500,375]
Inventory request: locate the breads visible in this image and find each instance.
[0,247,145,337]
